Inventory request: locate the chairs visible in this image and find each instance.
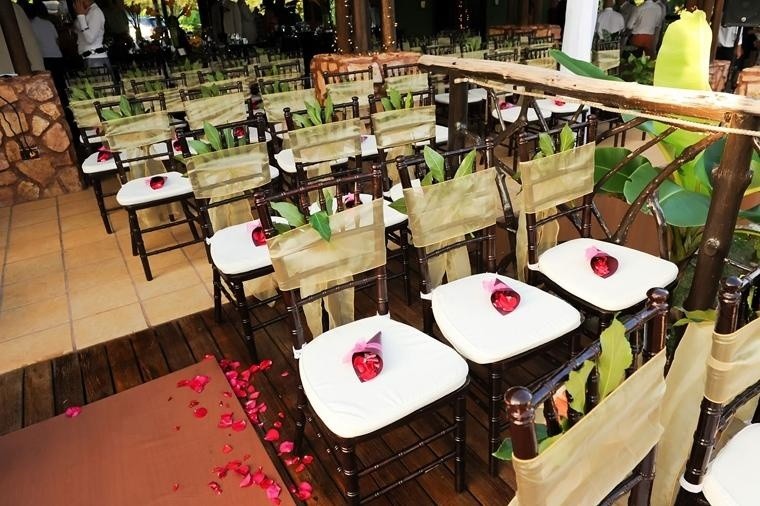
[253,162,471,505]
[396,135,586,479]
[60,36,648,353]
[671,266,760,505]
[520,114,679,337]
[501,286,669,505]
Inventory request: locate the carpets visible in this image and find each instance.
[0,356,296,504]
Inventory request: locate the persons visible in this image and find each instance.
[209,0,302,45]
[595,0,760,90]
[15,0,134,107]
